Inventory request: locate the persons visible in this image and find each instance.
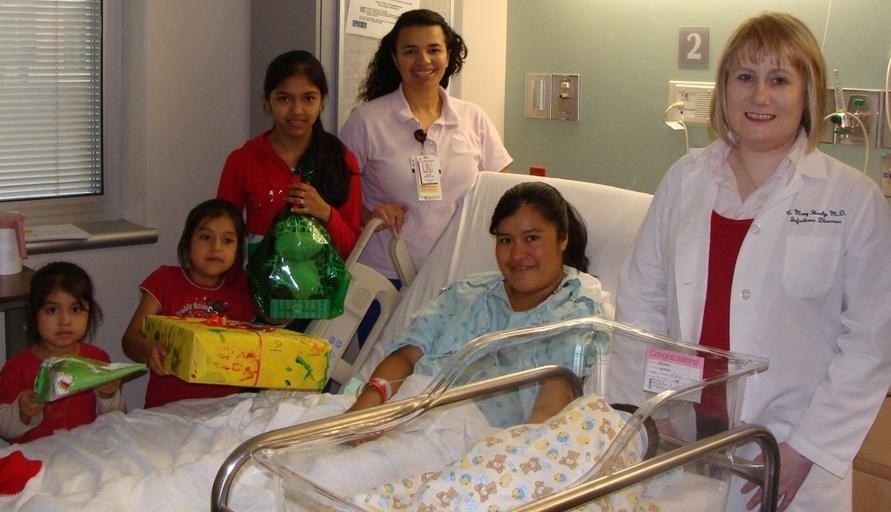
[122,198,271,409]
[214,50,364,396]
[337,10,515,351]
[338,182,612,447]
[0,261,124,444]
[613,11,890,512]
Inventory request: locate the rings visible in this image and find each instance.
[300,199,305,205]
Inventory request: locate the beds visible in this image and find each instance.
[0,172,656,512]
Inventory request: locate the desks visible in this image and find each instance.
[0,266,38,357]
